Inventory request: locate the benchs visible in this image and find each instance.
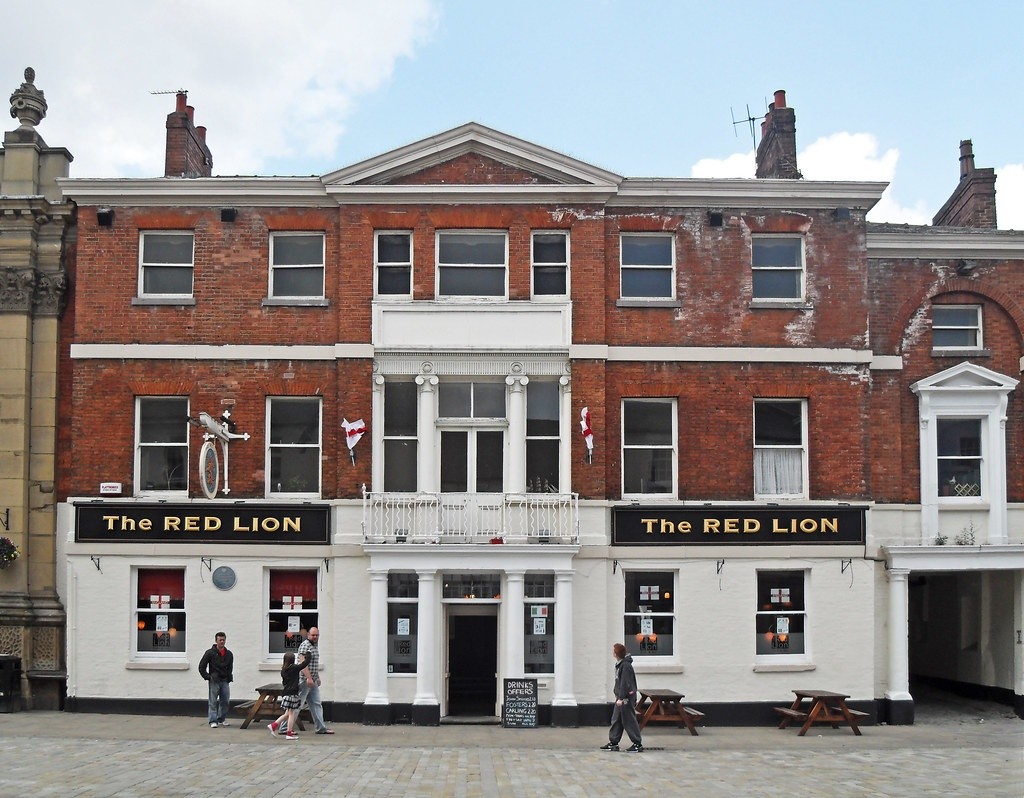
[832,707,870,720]
[683,706,705,721]
[773,707,808,722]
[234,700,257,715]
[635,709,642,720]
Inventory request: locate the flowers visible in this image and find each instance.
[0,537,22,570]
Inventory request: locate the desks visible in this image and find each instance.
[638,689,698,735]
[240,684,305,732]
[779,690,862,736]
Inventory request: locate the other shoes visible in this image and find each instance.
[278,728,298,735]
[316,728,335,734]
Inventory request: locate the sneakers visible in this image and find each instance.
[210,722,218,728]
[624,743,645,753]
[217,721,230,726]
[286,734,300,740]
[268,724,276,737]
[599,741,620,751]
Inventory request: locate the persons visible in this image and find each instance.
[199,632,234,728]
[600,642,643,753]
[267,652,311,740]
[279,626,335,735]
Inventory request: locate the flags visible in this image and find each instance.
[579,407,594,452]
[340,417,367,453]
